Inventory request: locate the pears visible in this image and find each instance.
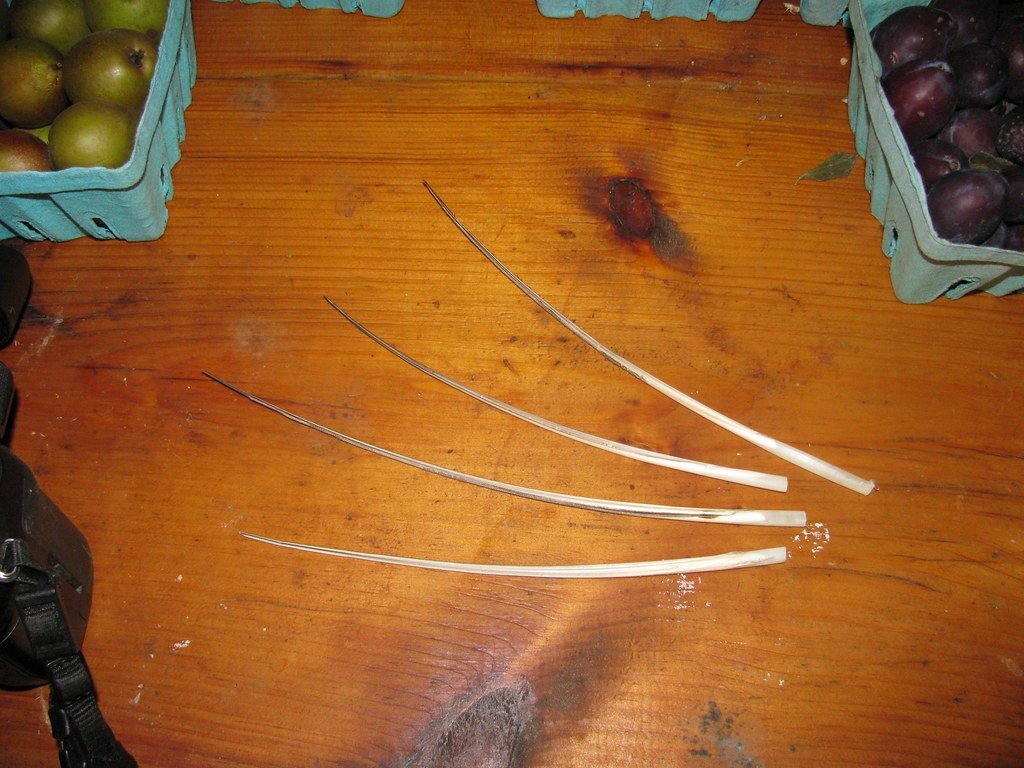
[0,0,169,172]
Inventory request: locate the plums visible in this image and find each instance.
[868,0,1024,260]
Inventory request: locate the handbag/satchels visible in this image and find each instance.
[0,449,138,768]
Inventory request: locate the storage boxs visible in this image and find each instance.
[536,0,761,22]
[800,0,1024,304]
[0,0,197,242]
